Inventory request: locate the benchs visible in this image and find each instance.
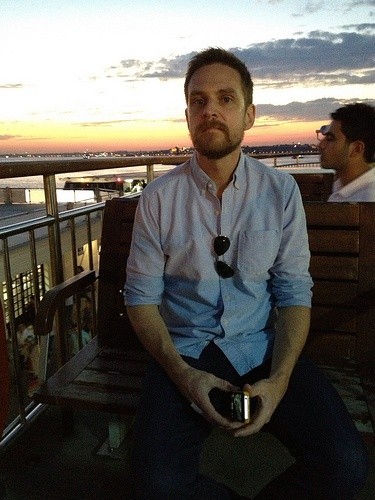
[33,197,374,455]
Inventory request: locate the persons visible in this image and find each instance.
[318,104,375,202]
[123,47,370,500]
[15,265,93,376]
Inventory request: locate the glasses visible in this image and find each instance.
[214,236,234,279]
[316,125,346,141]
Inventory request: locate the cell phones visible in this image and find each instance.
[224,391,250,423]
